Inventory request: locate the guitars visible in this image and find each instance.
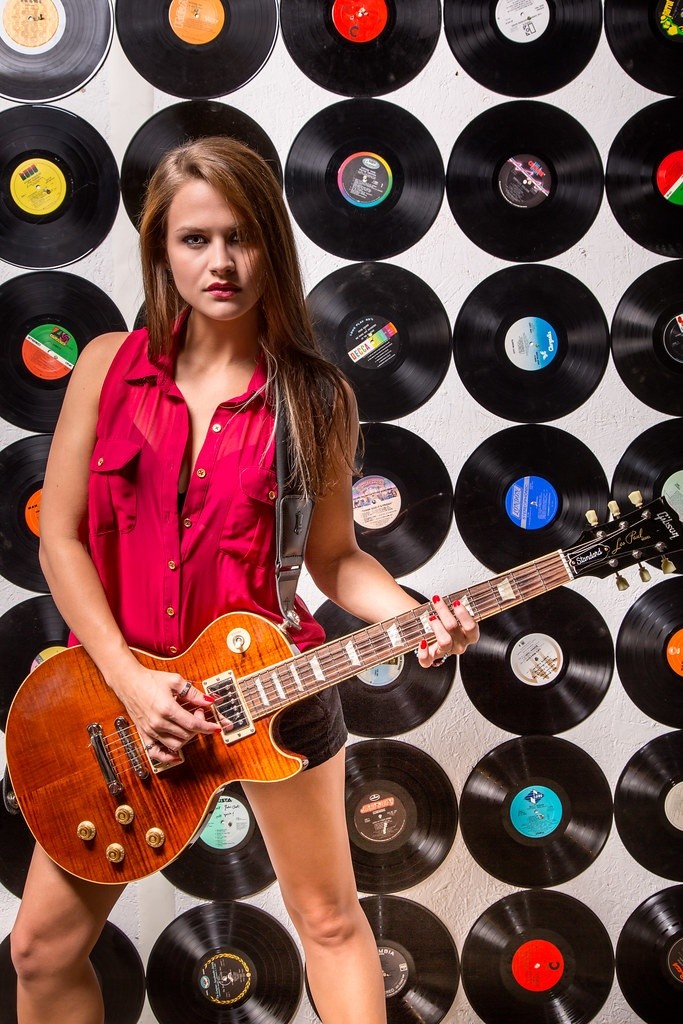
[3,487,683,887]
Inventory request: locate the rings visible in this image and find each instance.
[178,680,192,697]
[146,739,158,750]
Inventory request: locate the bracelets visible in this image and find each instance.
[414,647,448,666]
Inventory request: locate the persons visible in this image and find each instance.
[10,137,479,1024]
